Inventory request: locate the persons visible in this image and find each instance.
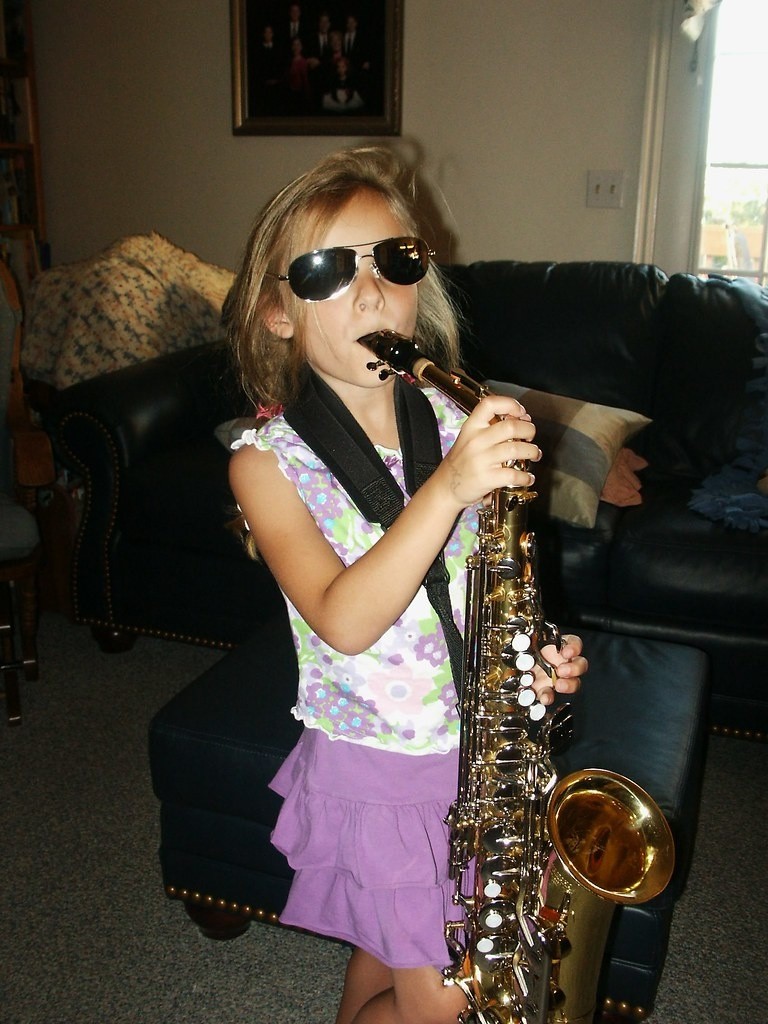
[222,146,545,1022]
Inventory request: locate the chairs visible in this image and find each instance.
[0,258,57,726]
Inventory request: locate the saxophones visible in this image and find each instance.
[356,330,676,1024]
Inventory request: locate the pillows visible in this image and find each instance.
[215,419,267,453]
[482,377,658,528]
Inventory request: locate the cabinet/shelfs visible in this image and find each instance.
[0,0,49,272]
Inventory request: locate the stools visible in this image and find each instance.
[147,626,713,1024]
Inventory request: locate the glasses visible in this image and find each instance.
[265,237,437,301]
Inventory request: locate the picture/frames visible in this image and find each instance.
[228,0,405,139]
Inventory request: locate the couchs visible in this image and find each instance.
[44,259,768,741]
